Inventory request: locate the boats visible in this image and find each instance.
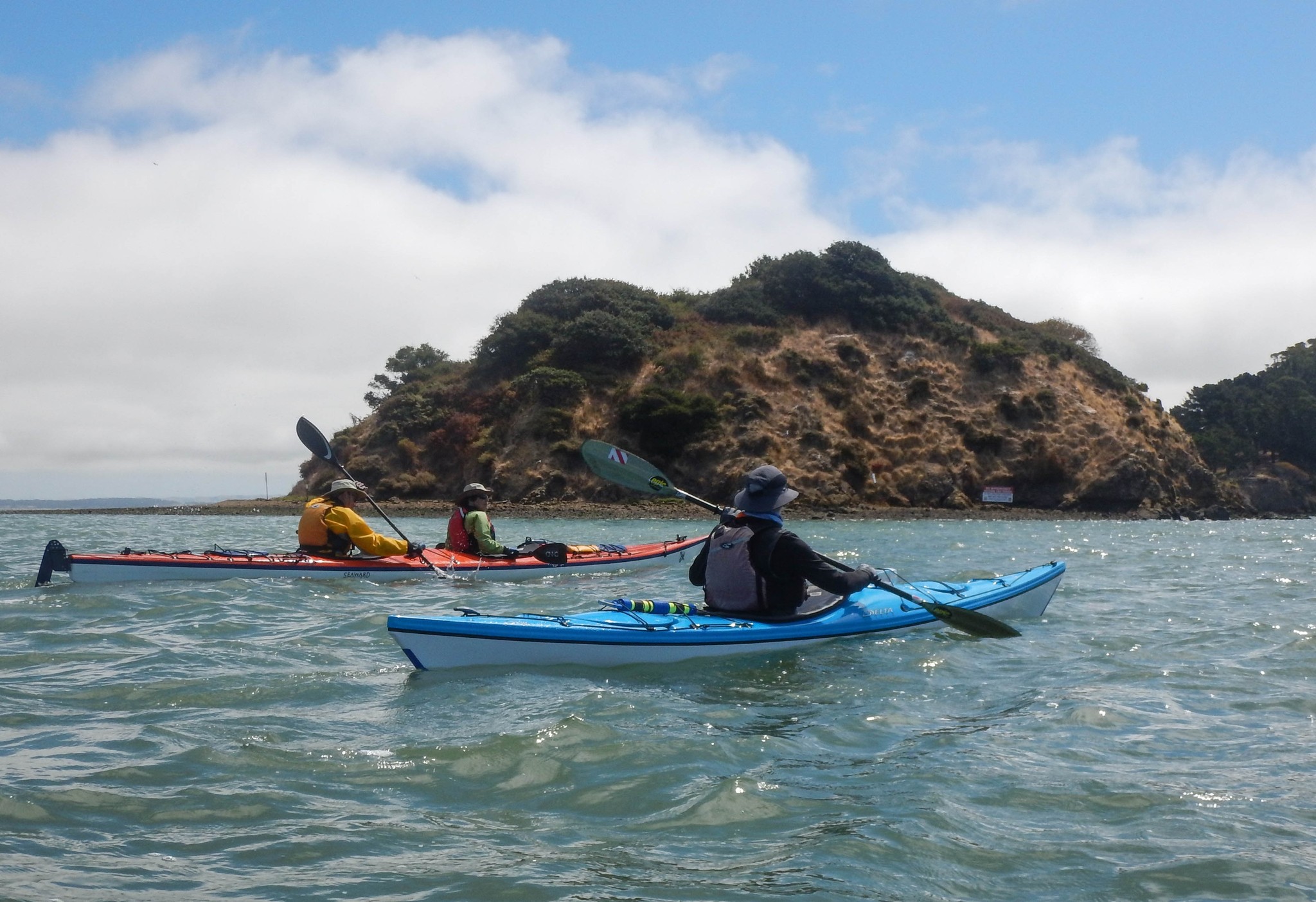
[33,533,711,590]
[386,558,1069,677]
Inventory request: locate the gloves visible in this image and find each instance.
[856,563,879,584]
[406,542,422,556]
[354,480,368,491]
[502,546,519,557]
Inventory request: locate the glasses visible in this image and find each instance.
[474,492,490,499]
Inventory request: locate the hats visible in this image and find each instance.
[454,483,494,506]
[734,465,799,512]
[321,479,367,501]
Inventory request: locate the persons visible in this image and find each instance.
[445,483,519,559]
[296,478,426,559]
[689,465,876,624]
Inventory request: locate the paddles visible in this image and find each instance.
[579,438,1024,638]
[295,414,451,578]
[435,542,568,565]
